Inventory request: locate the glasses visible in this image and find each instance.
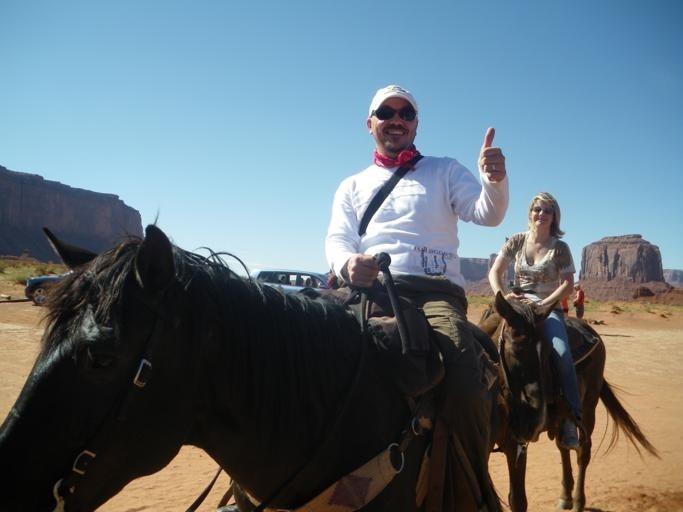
[532,206,554,214]
[376,105,416,122]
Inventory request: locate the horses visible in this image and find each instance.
[474,290,663,512]
[0,206,506,512]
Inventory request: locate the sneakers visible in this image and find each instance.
[559,420,579,448]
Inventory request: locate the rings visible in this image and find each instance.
[493,165,495,170]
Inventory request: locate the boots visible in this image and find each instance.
[453,388,490,466]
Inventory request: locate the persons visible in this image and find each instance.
[573,284,584,319]
[488,193,582,446]
[215,85,509,512]
[280,276,313,285]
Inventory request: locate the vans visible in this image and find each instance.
[251,270,329,295]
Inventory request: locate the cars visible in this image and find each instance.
[25,273,64,306]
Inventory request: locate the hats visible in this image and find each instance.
[368,85,417,117]
